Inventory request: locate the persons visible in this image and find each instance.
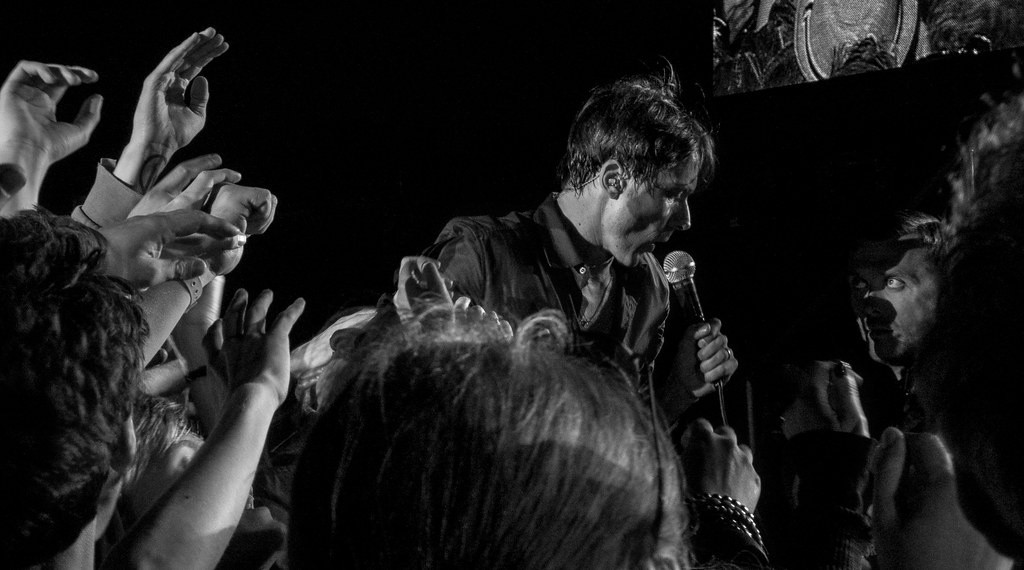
[1,27,1024,568]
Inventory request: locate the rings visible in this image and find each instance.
[724,348,731,360]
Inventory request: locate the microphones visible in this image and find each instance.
[663,250,724,388]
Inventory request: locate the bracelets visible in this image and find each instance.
[691,492,764,551]
[185,364,208,384]
[176,274,204,314]
